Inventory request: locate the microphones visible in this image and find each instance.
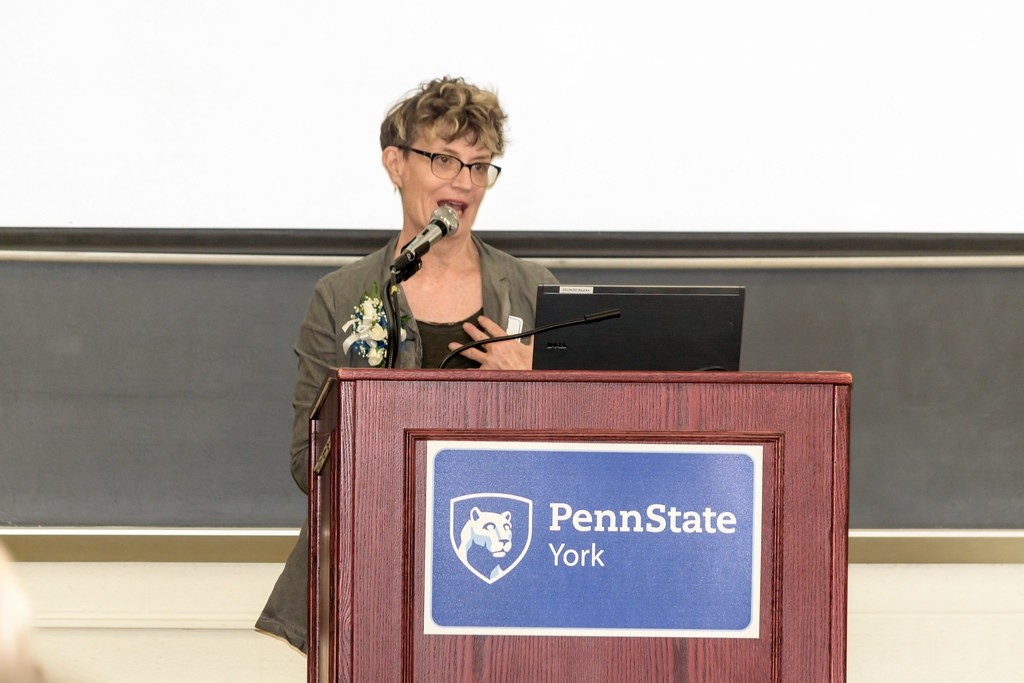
[439,308,623,370]
[391,204,460,272]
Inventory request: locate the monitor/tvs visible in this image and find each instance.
[533,285,746,372]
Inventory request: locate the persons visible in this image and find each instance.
[254,72,563,657]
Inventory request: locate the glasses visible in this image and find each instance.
[398,144,501,188]
